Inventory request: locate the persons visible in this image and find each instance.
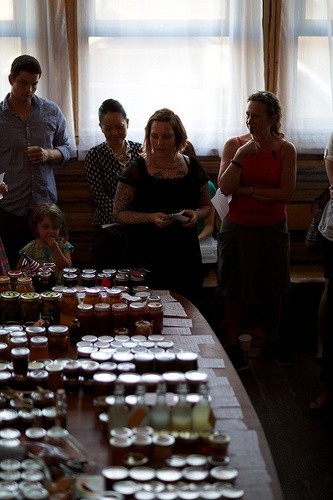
[179,143,222,280]
[17,203,74,285]
[83,99,146,271]
[298,132,333,418]
[0,55,71,273]
[113,110,212,313]
[215,94,296,366]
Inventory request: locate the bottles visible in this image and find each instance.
[107,383,215,434]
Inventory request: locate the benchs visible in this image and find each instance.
[56,154,326,290]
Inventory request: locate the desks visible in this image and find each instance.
[0,290,286,500]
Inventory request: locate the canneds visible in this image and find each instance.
[0,267,245,500]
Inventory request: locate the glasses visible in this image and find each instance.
[262,92,275,110]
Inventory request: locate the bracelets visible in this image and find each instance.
[196,208,201,218]
[230,159,243,168]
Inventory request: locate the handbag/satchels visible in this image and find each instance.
[305,185,330,248]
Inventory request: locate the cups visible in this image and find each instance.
[28,146,38,160]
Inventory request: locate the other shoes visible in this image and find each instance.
[309,390,333,410]
[223,341,238,350]
